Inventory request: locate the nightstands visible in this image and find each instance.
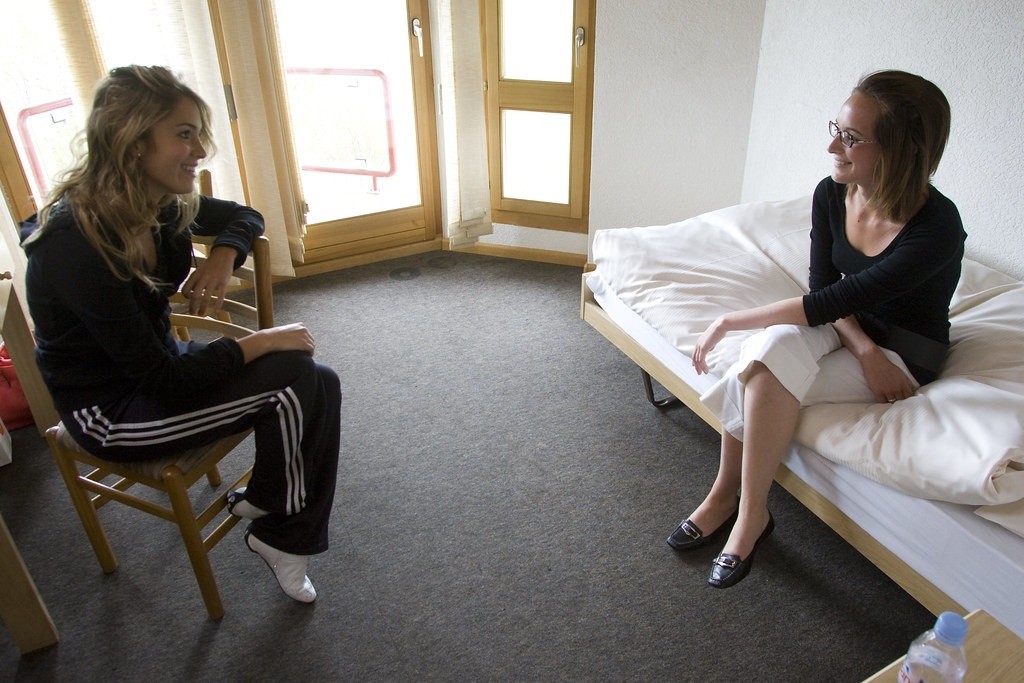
[861,609,1024,683]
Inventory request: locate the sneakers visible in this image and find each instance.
[245,531,317,602]
[227,487,269,521]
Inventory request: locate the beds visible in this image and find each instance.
[579,195,1024,641]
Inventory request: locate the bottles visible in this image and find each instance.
[898,613,967,683]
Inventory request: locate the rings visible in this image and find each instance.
[889,398,896,403]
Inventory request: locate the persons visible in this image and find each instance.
[21,66,341,604]
[668,69,966,587]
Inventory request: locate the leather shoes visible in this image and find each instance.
[707,504,777,589]
[666,497,743,552]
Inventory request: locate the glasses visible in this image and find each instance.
[827,121,881,148]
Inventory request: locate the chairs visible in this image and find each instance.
[43,227,305,622]
